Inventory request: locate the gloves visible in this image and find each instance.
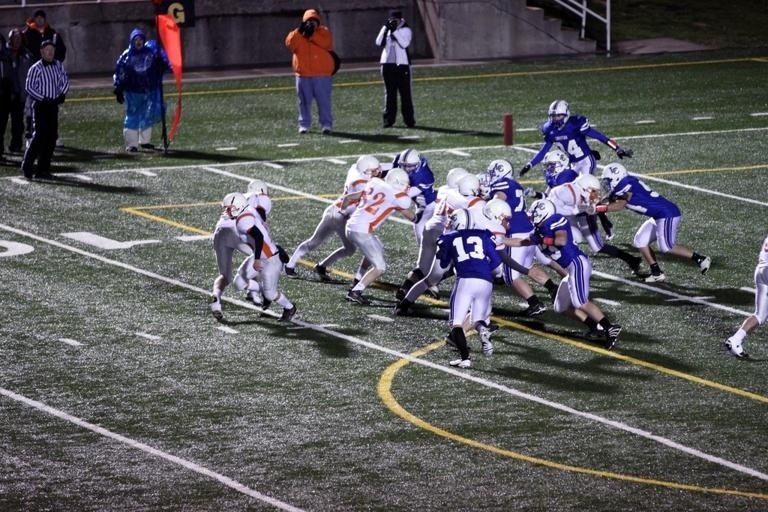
[616,148,634,160]
[519,163,532,177]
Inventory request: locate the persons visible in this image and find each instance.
[523,150,642,278]
[375,11,417,129]
[725,235,768,360]
[0,33,16,166]
[208,178,272,323]
[283,155,381,284]
[542,174,601,248]
[20,41,69,181]
[5,28,35,152]
[287,11,335,134]
[394,198,512,316]
[340,168,424,306]
[222,193,297,322]
[393,150,437,245]
[503,199,624,352]
[487,158,560,318]
[394,173,484,301]
[595,162,711,283]
[23,9,67,139]
[112,28,165,156]
[518,100,634,242]
[436,208,503,370]
[414,167,470,245]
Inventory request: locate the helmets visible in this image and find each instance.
[384,167,411,192]
[526,198,556,228]
[483,198,512,224]
[574,173,601,203]
[222,191,248,216]
[541,150,570,177]
[458,174,481,196]
[477,173,491,199]
[446,166,468,188]
[397,148,421,167]
[247,179,269,195]
[487,158,513,183]
[448,208,475,232]
[355,154,381,176]
[601,162,628,193]
[547,99,571,130]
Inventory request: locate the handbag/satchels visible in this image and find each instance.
[311,39,341,75]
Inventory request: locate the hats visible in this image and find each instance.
[389,10,402,19]
[40,38,55,49]
[33,9,46,18]
[8,28,25,39]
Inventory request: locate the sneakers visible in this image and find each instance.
[478,327,494,356]
[395,288,405,301]
[298,125,311,134]
[394,303,406,316]
[321,125,332,134]
[210,293,225,320]
[428,284,441,300]
[276,302,298,323]
[605,223,615,241]
[344,288,366,305]
[284,264,299,279]
[521,301,547,317]
[449,358,473,369]
[586,322,606,338]
[697,254,712,275]
[245,291,262,306]
[444,331,459,348]
[724,336,750,360]
[313,262,332,281]
[606,324,623,351]
[261,295,271,310]
[641,273,666,283]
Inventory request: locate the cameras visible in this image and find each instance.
[305,23,315,34]
[388,17,397,25]
